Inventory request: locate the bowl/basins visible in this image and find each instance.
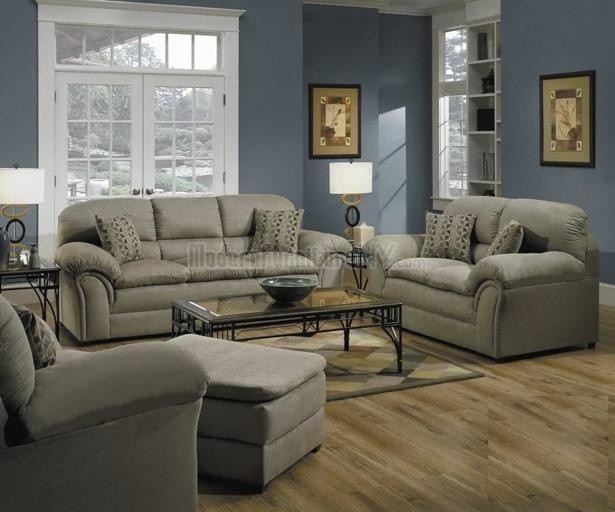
[259,276,319,301]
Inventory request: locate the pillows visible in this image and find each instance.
[486,216,527,260]
[6,299,58,370]
[415,207,481,266]
[240,204,308,256]
[92,209,149,268]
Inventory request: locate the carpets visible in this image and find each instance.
[113,311,486,405]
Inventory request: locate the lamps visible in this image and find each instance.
[0,161,50,273]
[324,156,376,250]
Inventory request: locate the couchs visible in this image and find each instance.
[48,192,355,348]
[362,190,601,365]
[0,291,211,511]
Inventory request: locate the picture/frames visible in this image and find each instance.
[306,81,363,161]
[535,68,597,171]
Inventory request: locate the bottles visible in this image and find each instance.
[29,243,40,269]
[477,32,488,60]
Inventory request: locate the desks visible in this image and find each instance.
[0,254,63,344]
[341,239,373,319]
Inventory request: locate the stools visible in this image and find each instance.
[158,331,330,495]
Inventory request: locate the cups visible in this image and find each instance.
[9,253,17,270]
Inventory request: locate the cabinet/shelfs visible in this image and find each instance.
[463,16,503,198]
